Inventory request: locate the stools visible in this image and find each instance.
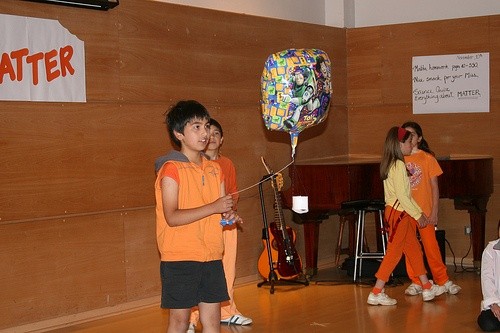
[334,211,370,269]
[340,199,395,284]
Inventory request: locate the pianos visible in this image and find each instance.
[273,153,495,279]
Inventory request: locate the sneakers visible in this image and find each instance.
[221,314,252,325]
[187,322,196,333]
[422,279,447,301]
[405,282,422,295]
[366,288,397,305]
[444,280,461,295]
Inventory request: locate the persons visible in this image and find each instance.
[186,118,253,333]
[477,222,500,333]
[153,99,244,333]
[401,122,462,296]
[365,126,446,305]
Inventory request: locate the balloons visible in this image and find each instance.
[260,49,333,158]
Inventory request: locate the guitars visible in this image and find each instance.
[257,172,304,280]
